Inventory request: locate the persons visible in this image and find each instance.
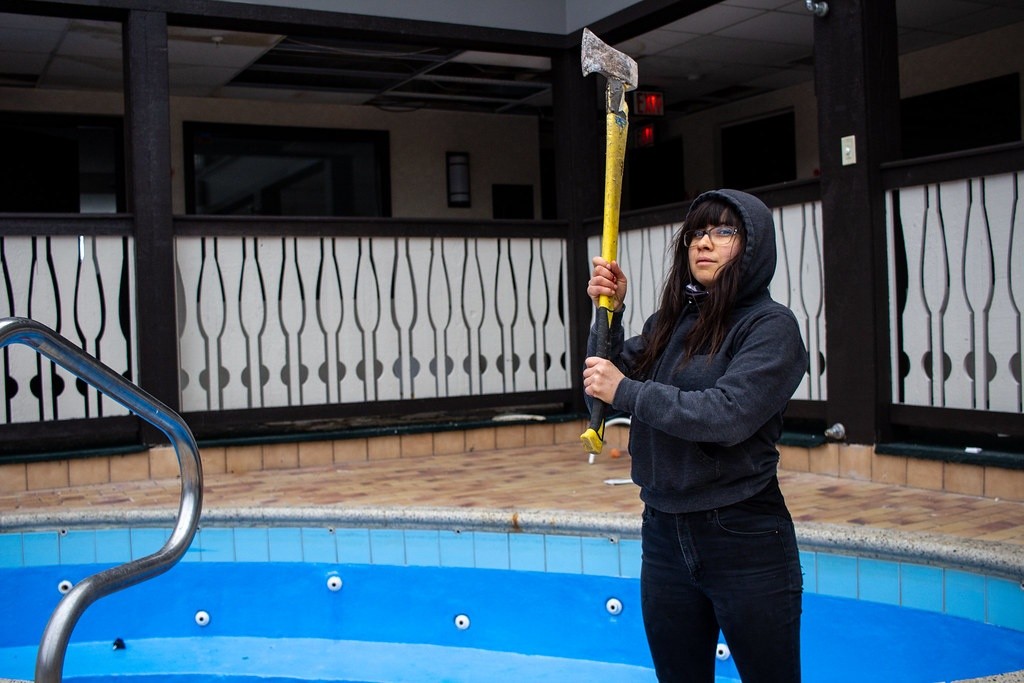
[582,188,805,683]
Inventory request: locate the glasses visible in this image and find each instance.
[684,224,745,247]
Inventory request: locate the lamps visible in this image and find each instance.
[444,150,473,208]
